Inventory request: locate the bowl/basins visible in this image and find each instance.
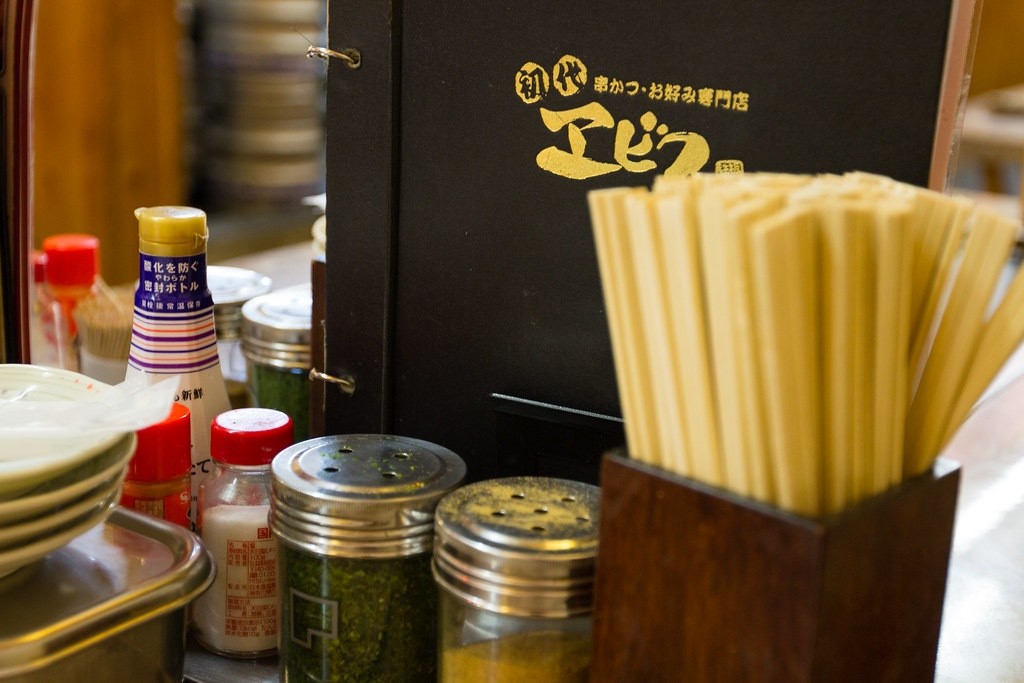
[0,359,137,580]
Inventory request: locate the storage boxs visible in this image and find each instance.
[1,497,217,683]
[590,447,963,683]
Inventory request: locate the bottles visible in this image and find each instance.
[269,433,463,683]
[193,405,292,658]
[437,480,600,683]
[204,263,315,444]
[122,402,198,535]
[202,3,326,200]
[29,231,99,378]
[125,205,236,495]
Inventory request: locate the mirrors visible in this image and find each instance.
[3,0,325,443]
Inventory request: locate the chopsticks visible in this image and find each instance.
[581,170,1024,523]
